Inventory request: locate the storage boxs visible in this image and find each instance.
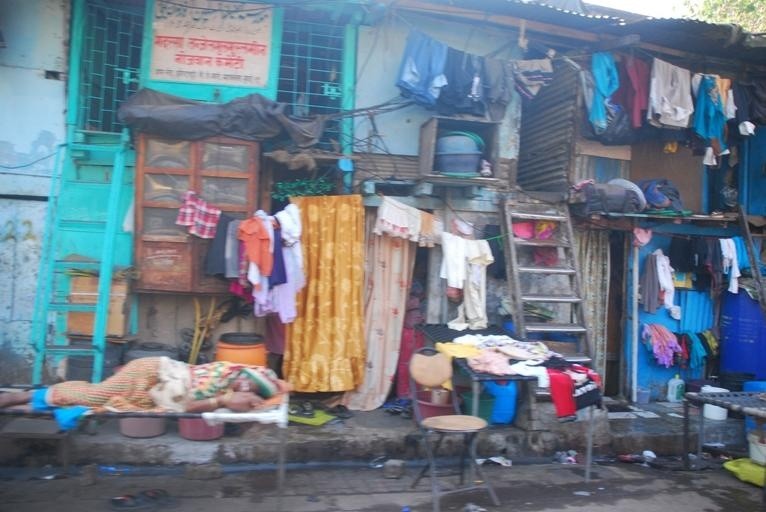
[66,275,131,340]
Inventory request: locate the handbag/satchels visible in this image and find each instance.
[571,182,641,221]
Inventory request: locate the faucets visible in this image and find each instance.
[216,333,271,370]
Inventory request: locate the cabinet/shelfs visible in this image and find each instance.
[134,131,261,297]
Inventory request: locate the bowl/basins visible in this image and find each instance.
[434,135,483,155]
[119,417,165,437]
[179,417,224,441]
[434,154,482,173]
[408,389,464,427]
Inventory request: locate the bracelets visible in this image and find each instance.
[208,397,218,409]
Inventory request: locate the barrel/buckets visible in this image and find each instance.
[122,340,181,365]
[464,392,495,429]
[482,379,518,425]
[669,375,687,405]
[746,431,765,465]
[702,386,729,422]
[742,381,766,428]
[720,283,765,383]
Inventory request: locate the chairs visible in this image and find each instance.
[408,347,501,512]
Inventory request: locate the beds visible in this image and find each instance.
[414,322,602,484]
[680,391,766,469]
[0,386,290,494]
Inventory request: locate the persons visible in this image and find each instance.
[0,356,278,433]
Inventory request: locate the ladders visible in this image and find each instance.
[737,204,766,310]
[501,201,607,430]
[31,128,131,384]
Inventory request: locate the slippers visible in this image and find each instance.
[323,403,353,419]
[110,495,156,511]
[387,406,402,415]
[288,404,314,417]
[401,406,414,419]
[139,488,181,508]
